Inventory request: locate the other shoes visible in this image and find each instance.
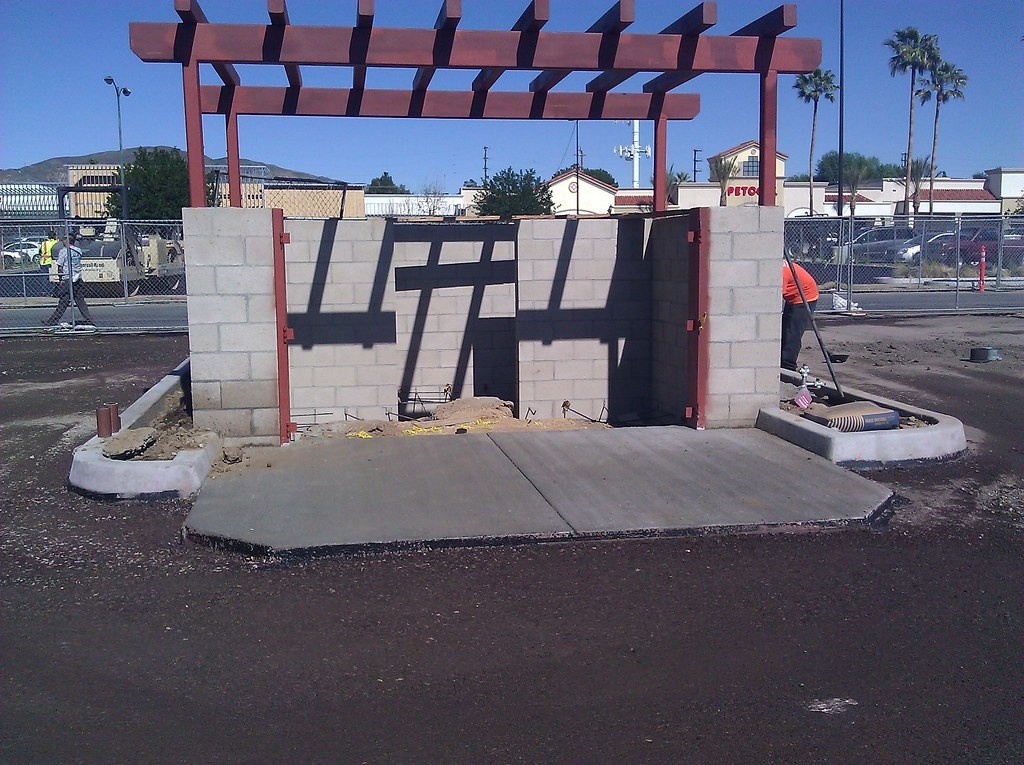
[41,319,55,326]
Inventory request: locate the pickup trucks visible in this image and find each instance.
[927,225,1024,267]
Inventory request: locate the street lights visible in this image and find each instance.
[103,75,133,216]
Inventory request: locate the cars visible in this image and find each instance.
[3,242,42,264]
[0,249,23,271]
[894,232,965,267]
[3,234,50,246]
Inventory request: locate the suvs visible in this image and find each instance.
[844,226,922,264]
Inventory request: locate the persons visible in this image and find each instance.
[780,259,819,372]
[41,234,96,325]
[40,231,59,270]
